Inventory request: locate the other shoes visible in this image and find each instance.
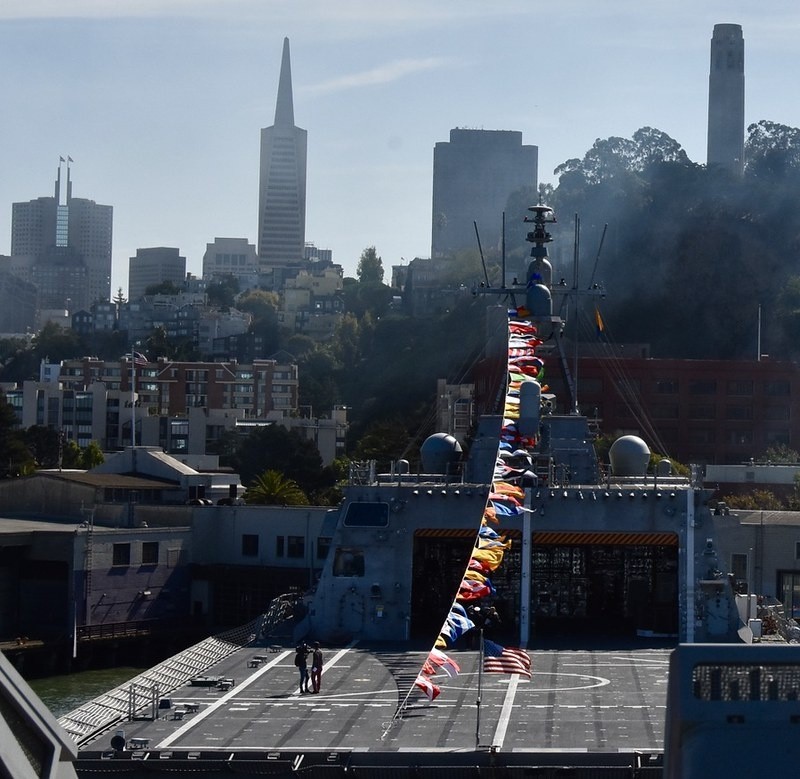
[311,690,319,694]
[305,689,311,693]
[300,690,305,693]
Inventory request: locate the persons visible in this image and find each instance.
[463,605,501,651]
[311,641,323,694]
[296,643,311,694]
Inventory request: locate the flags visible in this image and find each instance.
[134,351,148,366]
[482,639,532,679]
